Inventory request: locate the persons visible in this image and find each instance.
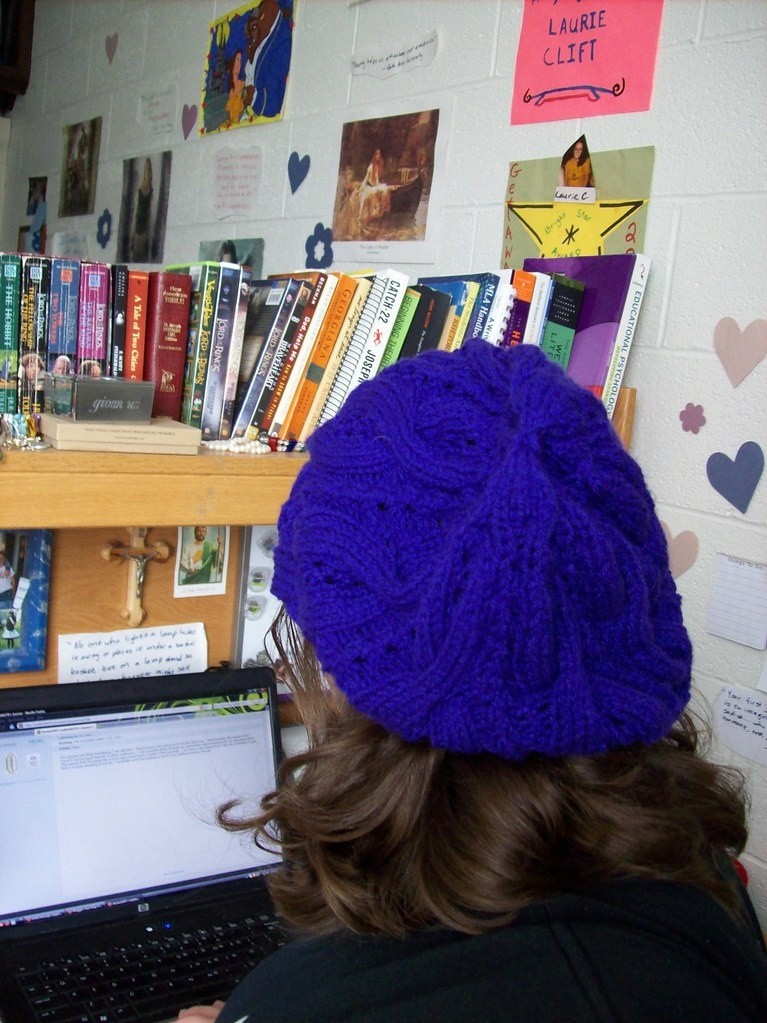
[216,241,238,263]
[169,336,767,1023]
[562,139,597,188]
[180,524,225,585]
[68,150,87,214]
[224,49,254,127]
[358,147,390,219]
[129,157,155,262]
[0,549,17,609]
[26,180,44,217]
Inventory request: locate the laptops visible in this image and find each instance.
[0,668,299,1022]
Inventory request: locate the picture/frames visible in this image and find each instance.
[57,116,102,215]
[197,238,263,282]
[316,94,458,264]
[197,0,297,136]
[114,150,173,264]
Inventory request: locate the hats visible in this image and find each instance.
[268,336,692,757]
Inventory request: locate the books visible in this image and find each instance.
[0,250,653,449]
